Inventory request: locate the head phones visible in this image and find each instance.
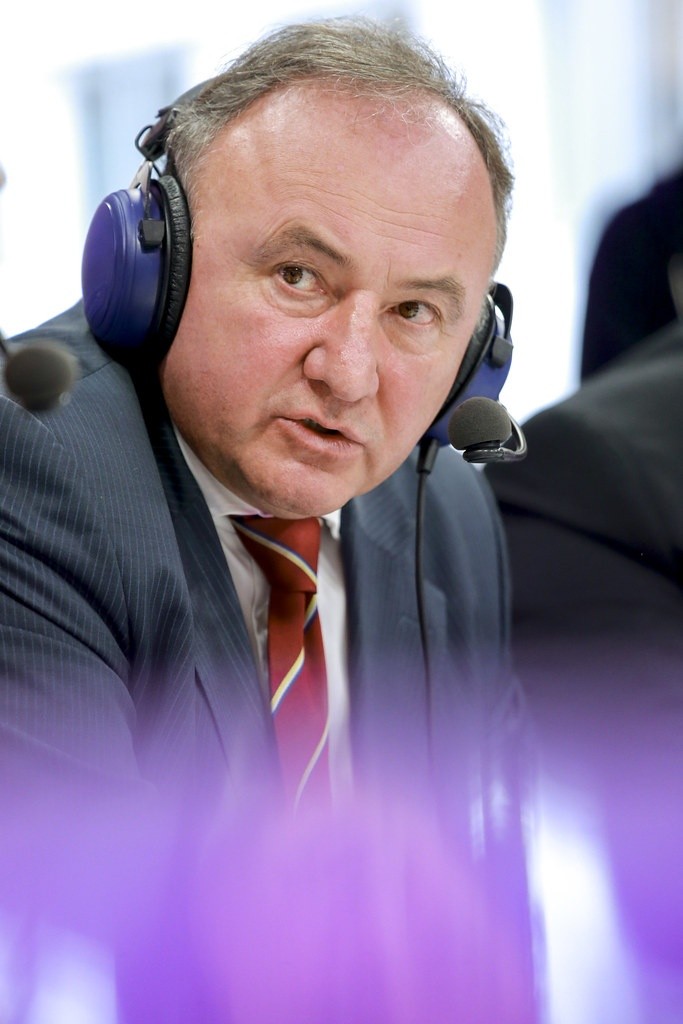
[81,74,529,473]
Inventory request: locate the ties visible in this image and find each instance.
[230,503,335,847]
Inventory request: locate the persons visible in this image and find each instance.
[481,159,683,1024]
[0,15,511,1024]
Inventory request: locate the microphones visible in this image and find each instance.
[448,397,529,463]
[0,333,75,414]
